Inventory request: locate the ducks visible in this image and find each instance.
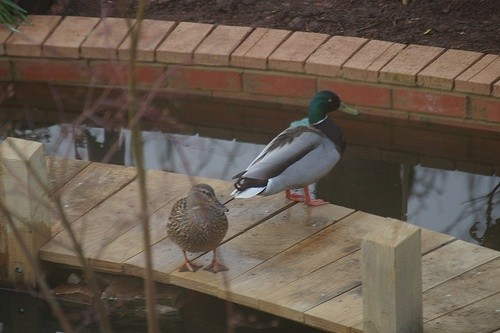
[166,184,230,275]
[229,90,361,207]
